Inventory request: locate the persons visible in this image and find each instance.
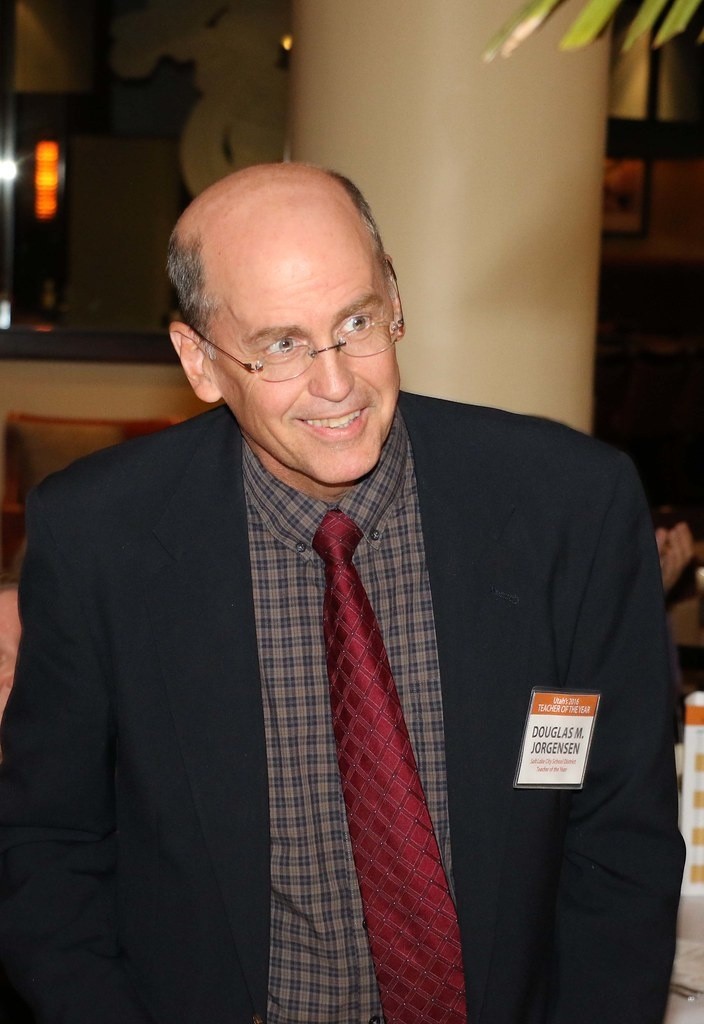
[0,164,690,1024]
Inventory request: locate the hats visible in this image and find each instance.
[181,84,289,198]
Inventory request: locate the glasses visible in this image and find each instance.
[192,260,405,382]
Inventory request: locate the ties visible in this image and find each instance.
[313,511,467,1024]
